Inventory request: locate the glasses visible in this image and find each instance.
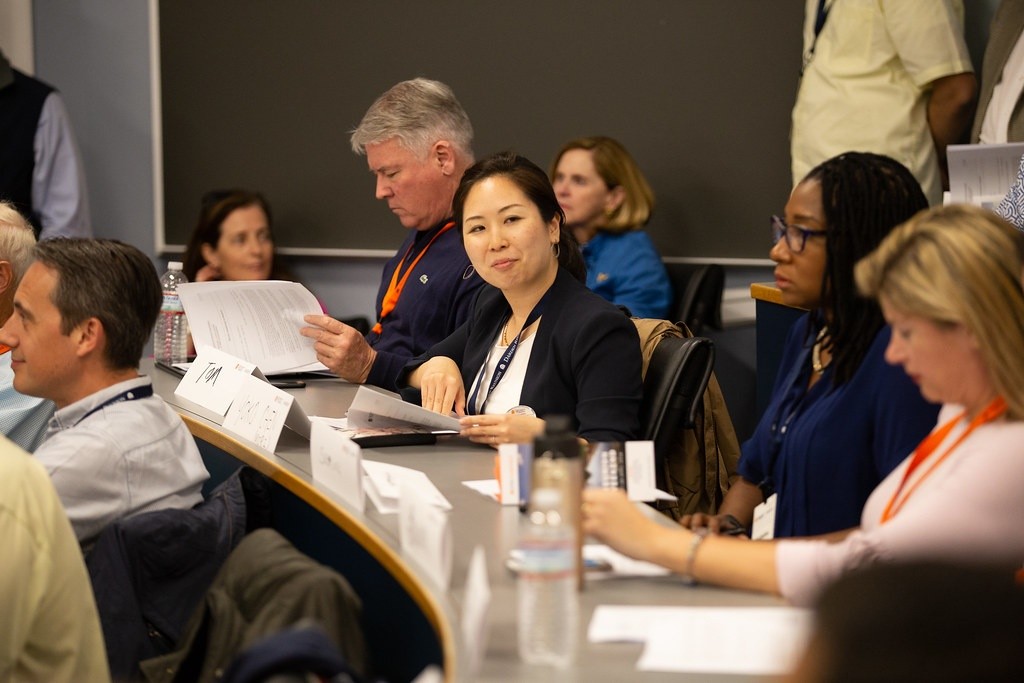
[769,215,828,255]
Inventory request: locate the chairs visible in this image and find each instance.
[670,266,726,332]
[640,337,717,510]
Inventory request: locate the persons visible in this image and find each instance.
[394,150,645,459]
[0,434,108,683]
[0,203,58,454]
[790,0,976,209]
[580,205,1024,605]
[0,235,211,519]
[789,548,1024,683]
[298,77,488,394]
[549,135,671,320]
[183,188,330,356]
[678,152,944,547]
[0,47,95,240]
[964,0,1024,144]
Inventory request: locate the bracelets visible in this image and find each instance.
[687,528,711,573]
[719,513,744,528]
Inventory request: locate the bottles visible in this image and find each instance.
[153,262,188,364]
[530,434,587,592]
[515,488,582,667]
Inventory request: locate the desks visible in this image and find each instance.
[140,356,813,683]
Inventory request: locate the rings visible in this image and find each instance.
[493,436,495,444]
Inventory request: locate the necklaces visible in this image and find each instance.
[504,324,510,346]
[812,327,824,374]
